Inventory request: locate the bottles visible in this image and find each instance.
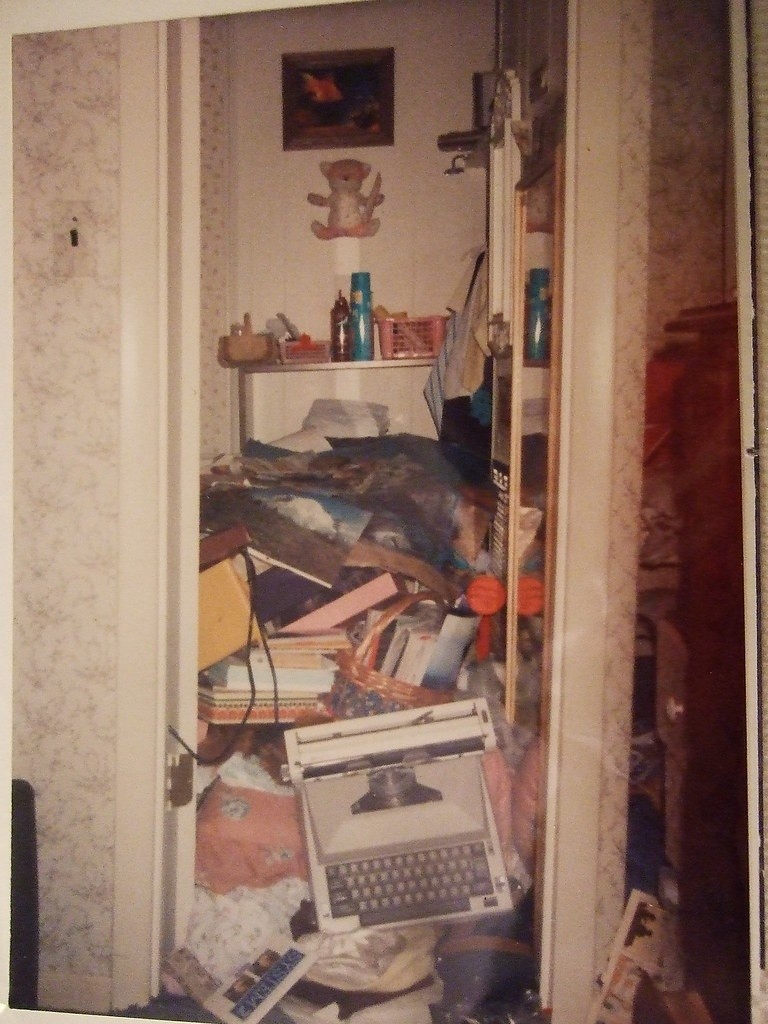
[525,268,551,361]
[330,298,351,362]
[349,272,375,362]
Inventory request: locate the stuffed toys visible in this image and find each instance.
[307,158,385,240]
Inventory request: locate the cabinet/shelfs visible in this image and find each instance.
[238,359,437,448]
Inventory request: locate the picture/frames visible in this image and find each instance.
[280,47,398,152]
